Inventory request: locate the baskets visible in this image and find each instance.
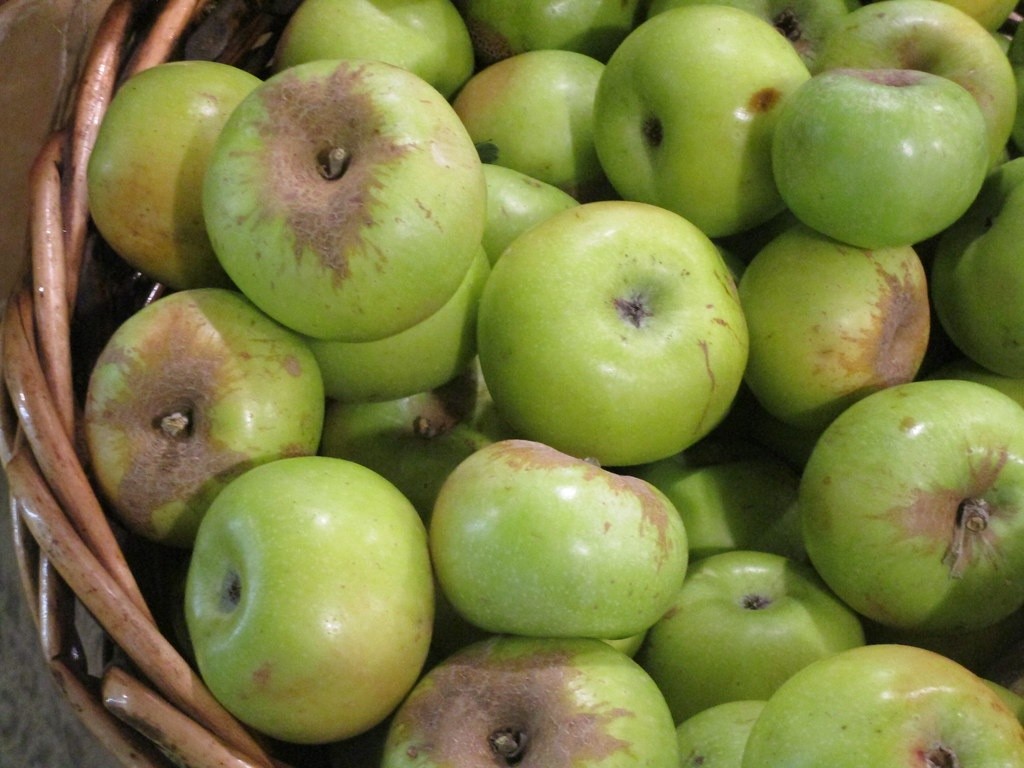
[0,0,1024,768]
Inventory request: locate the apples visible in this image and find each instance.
[82,0,1024,768]
